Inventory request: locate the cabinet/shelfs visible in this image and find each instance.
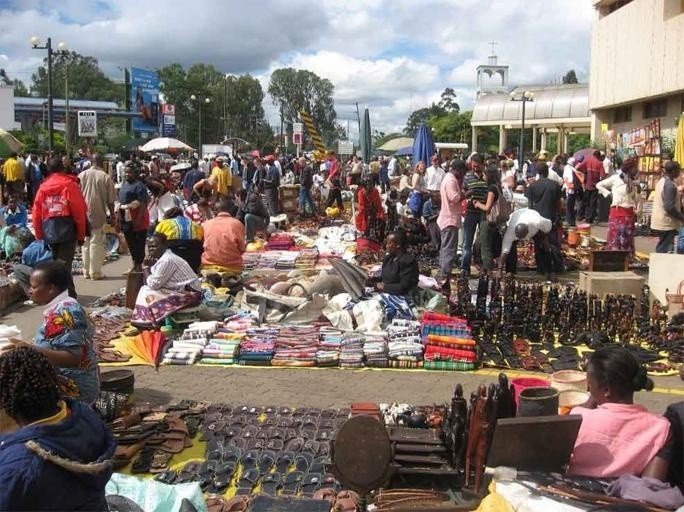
[617,118,660,202]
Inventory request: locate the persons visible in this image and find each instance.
[32,159,86,274]
[262,154,280,230]
[356,177,385,240]
[154,208,204,274]
[0,196,35,266]
[364,232,419,308]
[641,363,684,496]
[650,160,684,253]
[0,143,624,228]
[595,159,640,264]
[234,188,270,243]
[76,153,120,280]
[116,162,152,274]
[131,233,205,333]
[568,345,670,477]
[575,150,604,223]
[322,150,345,217]
[498,210,554,281]
[524,163,568,274]
[436,159,473,278]
[1,260,101,407]
[0,345,117,512]
[198,200,246,288]
[2,151,24,205]
[473,167,503,276]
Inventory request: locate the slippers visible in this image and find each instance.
[164,399,247,436]
[111,349,132,359]
[336,489,358,512]
[284,427,296,442]
[321,408,335,418]
[113,411,141,429]
[337,407,350,418]
[291,415,302,426]
[286,437,304,453]
[308,456,326,474]
[205,495,222,512]
[199,460,214,481]
[215,461,235,485]
[267,438,284,451]
[244,418,262,438]
[323,473,337,488]
[302,439,320,455]
[298,428,315,439]
[318,442,330,457]
[130,445,154,473]
[222,494,247,512]
[271,427,285,438]
[207,434,226,446]
[336,417,346,429]
[118,430,154,443]
[235,469,259,495]
[126,419,158,432]
[256,428,268,439]
[223,445,239,463]
[278,406,290,416]
[308,406,320,416]
[248,438,266,450]
[160,431,185,453]
[145,432,166,444]
[292,407,306,417]
[102,354,129,362]
[154,449,173,463]
[313,487,335,503]
[260,472,279,496]
[315,429,331,440]
[239,449,258,469]
[258,450,276,473]
[264,405,277,416]
[319,418,334,429]
[90,314,132,349]
[249,405,263,417]
[282,471,303,496]
[275,451,293,474]
[300,474,321,495]
[203,445,223,460]
[279,417,290,427]
[294,452,313,472]
[200,420,231,441]
[150,464,169,473]
[180,460,202,483]
[304,416,317,428]
[262,416,277,429]
[230,436,247,449]
[209,481,226,495]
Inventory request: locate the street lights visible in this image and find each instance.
[191,90,210,159]
[31,36,71,158]
[509,89,534,172]
